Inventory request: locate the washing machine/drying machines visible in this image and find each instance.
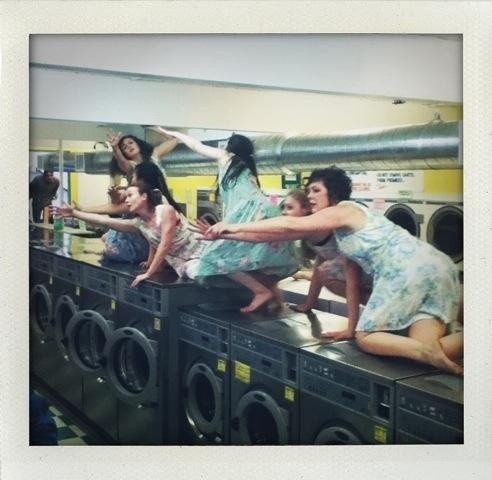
[28,223,462,448]
[195,189,462,276]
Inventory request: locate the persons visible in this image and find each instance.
[29,168,59,224]
[46,181,208,290]
[145,124,303,313]
[205,163,464,377]
[187,189,373,341]
[72,129,180,262]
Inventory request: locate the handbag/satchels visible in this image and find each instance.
[104,228,149,265]
